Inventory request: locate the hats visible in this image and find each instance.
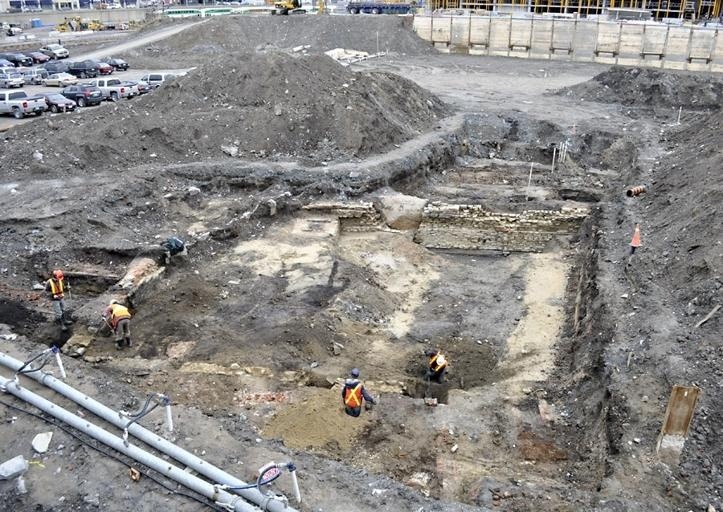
[352,368,359,376]
[110,299,117,304]
[436,355,446,365]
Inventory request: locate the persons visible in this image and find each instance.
[102,299,132,350]
[45,269,72,331]
[425,349,447,383]
[342,368,377,417]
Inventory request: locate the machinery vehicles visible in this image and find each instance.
[345,0,412,15]
[269,0,325,16]
[55,14,85,33]
[86,18,107,32]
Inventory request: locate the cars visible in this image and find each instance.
[0,41,188,122]
[3,6,44,36]
[96,0,242,31]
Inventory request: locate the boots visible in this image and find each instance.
[115,336,132,350]
[59,314,73,332]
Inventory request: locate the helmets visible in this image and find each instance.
[53,271,58,275]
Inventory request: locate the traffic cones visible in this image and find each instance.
[624,222,647,251]
